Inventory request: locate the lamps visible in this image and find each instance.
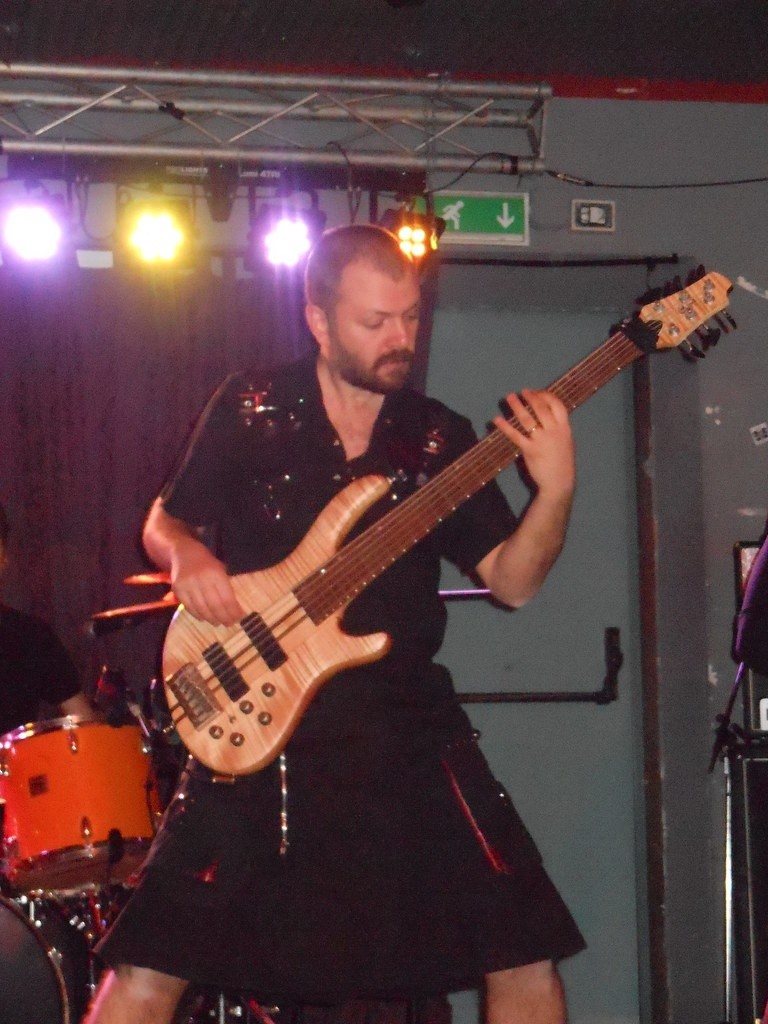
[245,182,327,277]
[1,174,68,269]
[370,185,445,275]
[118,181,195,275]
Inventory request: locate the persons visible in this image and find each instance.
[78,227,588,1023]
[0,605,92,729]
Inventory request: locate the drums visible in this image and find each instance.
[0,709,458,1024]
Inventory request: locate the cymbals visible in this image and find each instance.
[72,570,183,637]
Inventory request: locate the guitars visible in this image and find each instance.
[149,259,740,780]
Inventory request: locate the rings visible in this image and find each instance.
[527,424,539,436]
